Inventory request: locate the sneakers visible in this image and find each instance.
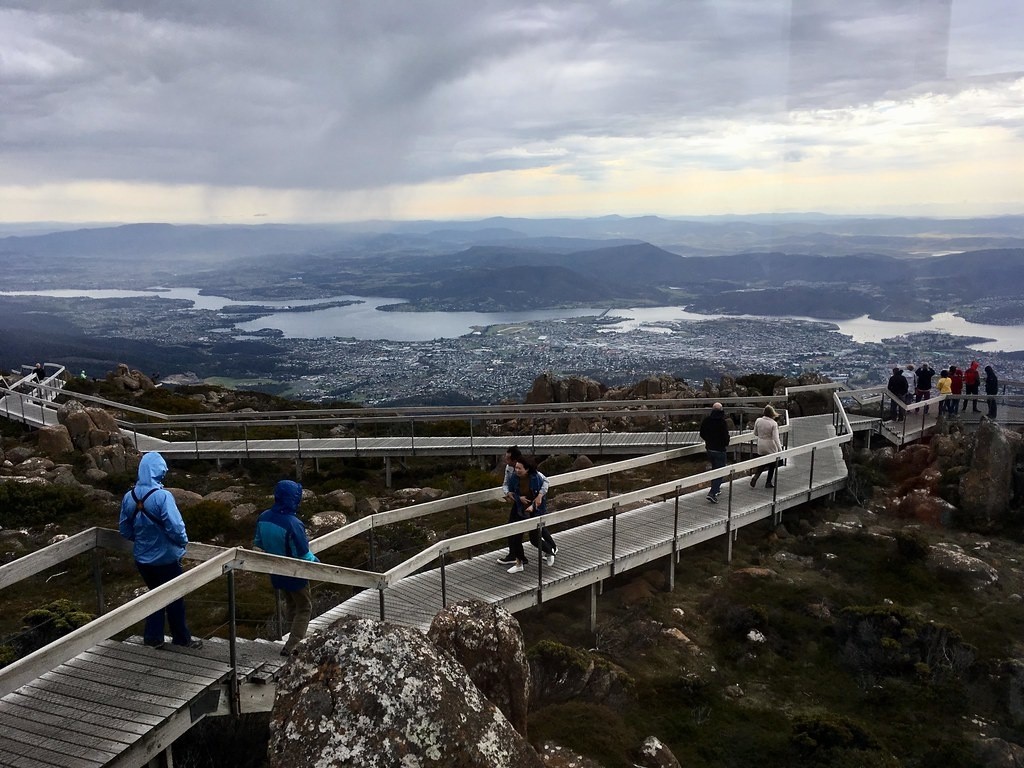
[715,491,721,496]
[542,546,558,560]
[497,557,517,564]
[547,548,554,566]
[706,492,718,503]
[507,565,524,573]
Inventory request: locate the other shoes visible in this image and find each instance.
[279,648,298,656]
[943,415,947,419]
[765,482,775,488]
[153,642,166,649]
[961,408,967,412]
[894,418,898,423]
[925,412,928,415]
[175,638,204,650]
[954,414,961,418]
[973,410,982,413]
[936,416,942,419]
[900,416,905,424]
[750,478,756,487]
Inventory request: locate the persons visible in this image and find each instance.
[496,446,559,573]
[254,480,321,657]
[81,370,87,379]
[937,366,964,418]
[33,363,45,398]
[888,362,936,423]
[960,361,982,413]
[700,402,730,503]
[750,405,782,488]
[119,452,203,650]
[151,371,160,384]
[983,366,998,420]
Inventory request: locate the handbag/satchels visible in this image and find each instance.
[914,387,919,395]
